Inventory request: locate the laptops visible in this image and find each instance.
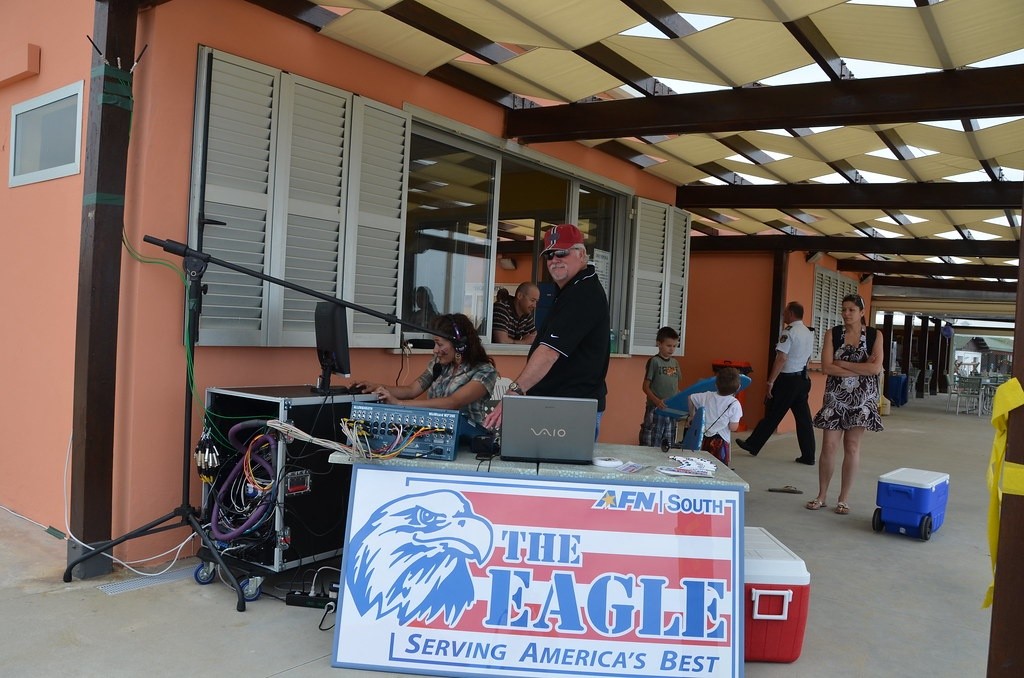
[501,395,598,465]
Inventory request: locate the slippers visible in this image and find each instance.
[807,498,827,510]
[834,501,849,515]
[768,485,803,494]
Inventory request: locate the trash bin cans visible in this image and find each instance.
[887,372,908,408]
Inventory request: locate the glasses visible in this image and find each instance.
[544,247,579,260]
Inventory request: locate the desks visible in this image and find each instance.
[325,448,749,678]
[956,380,1001,414]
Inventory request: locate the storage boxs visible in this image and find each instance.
[194,386,381,600]
[742,525,811,665]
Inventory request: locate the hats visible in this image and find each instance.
[540,223,586,260]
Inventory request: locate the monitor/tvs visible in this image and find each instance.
[310,302,351,395]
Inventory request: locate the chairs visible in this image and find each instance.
[946,371,1012,417]
[895,367,934,398]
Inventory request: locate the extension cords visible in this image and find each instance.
[286,591,337,611]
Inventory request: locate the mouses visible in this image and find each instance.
[348,383,372,394]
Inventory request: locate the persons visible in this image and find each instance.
[483,224,611,444]
[803,294,884,515]
[735,301,816,465]
[685,367,743,466]
[492,281,540,345]
[639,326,682,447]
[348,313,498,425]
[409,286,437,331]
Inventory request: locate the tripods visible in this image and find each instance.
[64,257,246,612]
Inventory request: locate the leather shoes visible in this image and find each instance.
[796,457,815,465]
[736,438,758,456]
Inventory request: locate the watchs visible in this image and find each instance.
[509,382,524,396]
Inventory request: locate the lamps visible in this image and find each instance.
[806,251,825,264]
[859,273,875,286]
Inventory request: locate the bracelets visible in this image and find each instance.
[765,381,774,386]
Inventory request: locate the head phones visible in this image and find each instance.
[447,314,467,352]
